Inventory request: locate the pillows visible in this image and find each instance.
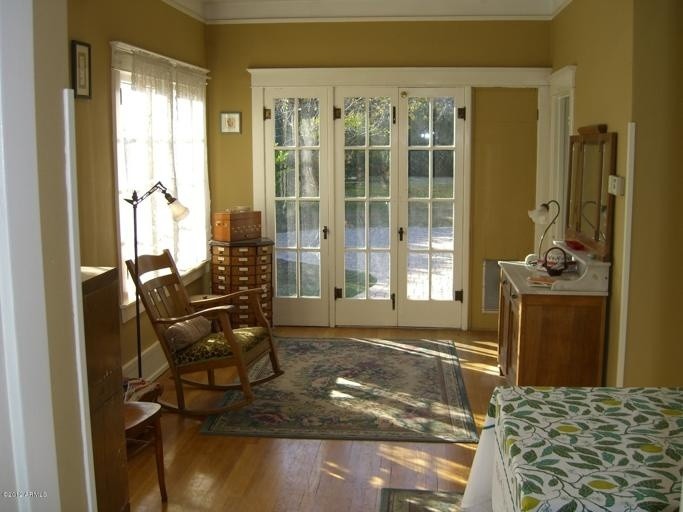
[166,316,212,353]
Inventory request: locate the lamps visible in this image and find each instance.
[526,200,560,271]
[124,180,189,380]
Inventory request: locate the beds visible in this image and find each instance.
[458,386,683,512]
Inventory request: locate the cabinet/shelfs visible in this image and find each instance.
[209,237,275,331]
[496,241,610,386]
[82,264,131,512]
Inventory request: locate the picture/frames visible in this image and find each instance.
[71,40,92,99]
[219,111,242,134]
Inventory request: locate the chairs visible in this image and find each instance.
[126,249,284,417]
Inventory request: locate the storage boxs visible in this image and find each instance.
[212,211,261,242]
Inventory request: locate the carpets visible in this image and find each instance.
[198,332,479,443]
[379,487,470,512]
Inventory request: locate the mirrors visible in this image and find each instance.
[565,124,617,262]
